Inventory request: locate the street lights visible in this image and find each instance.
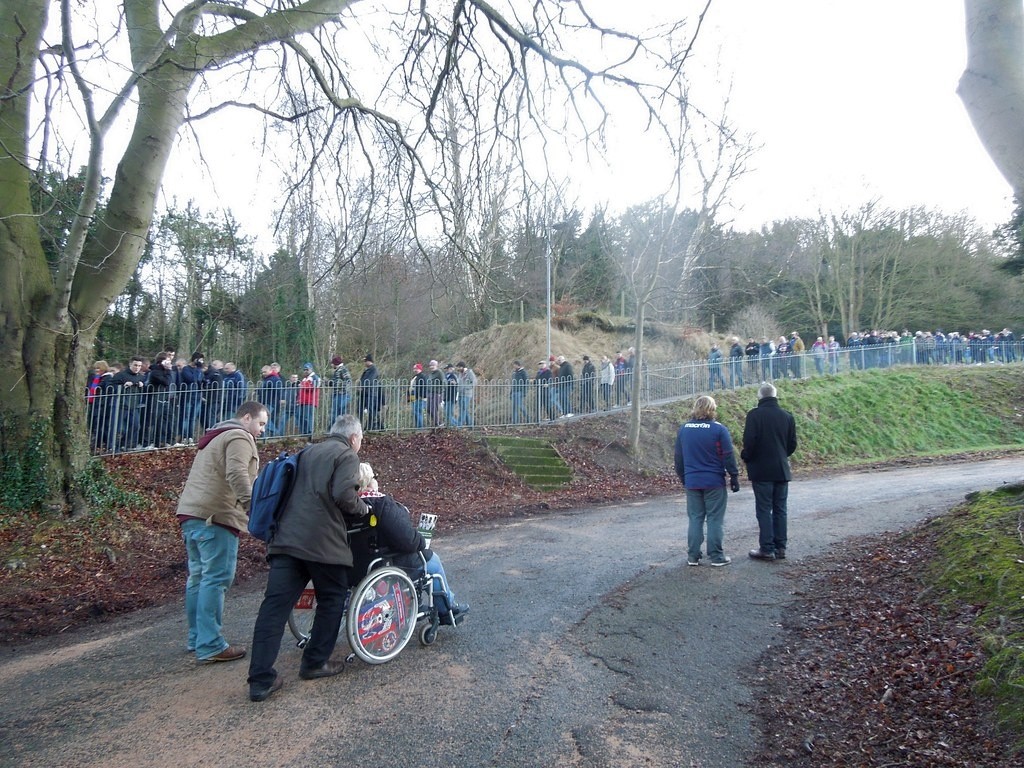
[545,221,568,369]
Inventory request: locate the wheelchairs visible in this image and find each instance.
[287,505,465,666]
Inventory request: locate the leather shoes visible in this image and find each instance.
[419,604,431,612]
[748,547,785,561]
[451,603,470,615]
[204,645,247,661]
[300,660,345,679]
[250,675,284,701]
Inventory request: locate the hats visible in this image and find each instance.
[332,357,343,365]
[364,354,372,361]
[304,363,313,368]
[760,383,775,397]
[852,327,1011,342]
[790,332,799,335]
[414,360,465,369]
[512,346,635,365]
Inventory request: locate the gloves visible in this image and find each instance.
[195,358,202,367]
[163,362,172,369]
[729,475,740,493]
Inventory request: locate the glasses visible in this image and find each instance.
[303,369,311,371]
[364,360,369,362]
[372,472,378,480]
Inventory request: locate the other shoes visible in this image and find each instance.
[545,401,633,420]
[939,358,1018,365]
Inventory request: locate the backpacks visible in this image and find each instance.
[249,442,314,543]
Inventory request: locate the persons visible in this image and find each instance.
[407,360,478,428]
[247,414,374,701]
[673,396,740,566]
[846,328,1024,371]
[741,383,797,561]
[707,332,840,389]
[84,347,385,452]
[344,463,469,621]
[510,347,635,423]
[175,402,270,661]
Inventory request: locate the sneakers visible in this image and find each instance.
[687,557,732,566]
